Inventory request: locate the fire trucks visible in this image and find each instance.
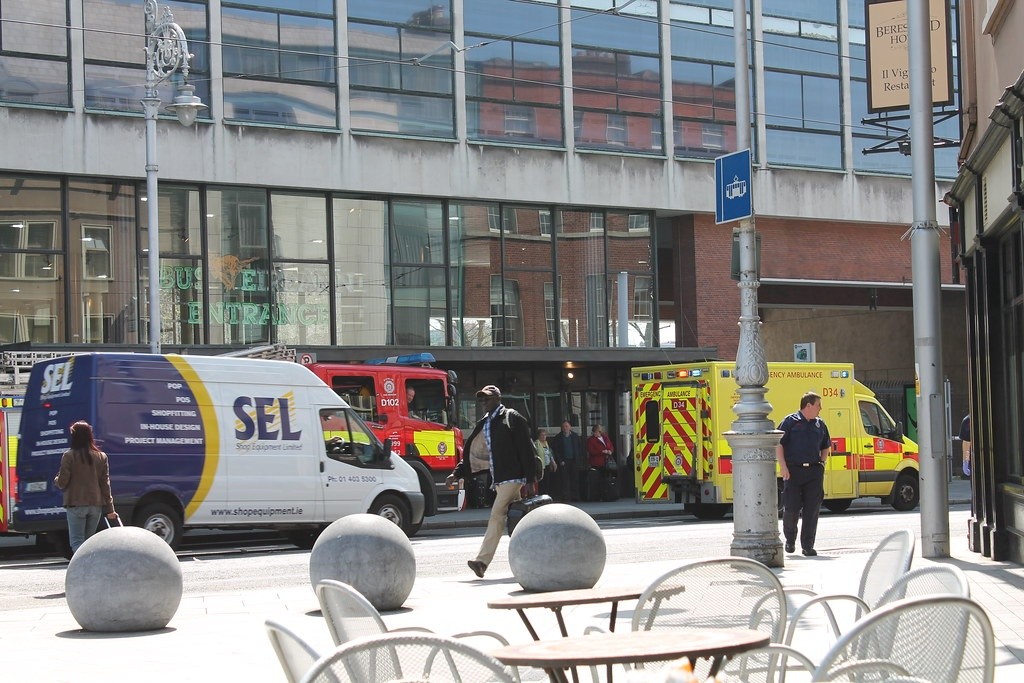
[0,342,468,552]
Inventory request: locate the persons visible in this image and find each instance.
[53,422,117,555]
[588,424,613,469]
[533,428,558,496]
[444,384,536,577]
[325,437,342,446]
[959,414,974,517]
[775,392,831,556]
[553,421,582,502]
[406,385,422,419]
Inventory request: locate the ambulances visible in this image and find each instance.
[631,363,920,521]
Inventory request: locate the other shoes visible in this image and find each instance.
[468,560,486,577]
[785,542,795,553]
[802,548,817,556]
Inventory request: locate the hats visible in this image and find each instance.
[476,384,501,397]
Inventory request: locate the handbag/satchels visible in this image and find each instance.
[604,454,618,477]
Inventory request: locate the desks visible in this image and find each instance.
[489,585,686,682]
[495,627,770,683]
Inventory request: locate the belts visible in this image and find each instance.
[791,463,819,466]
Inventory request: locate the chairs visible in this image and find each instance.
[266,530,996,682]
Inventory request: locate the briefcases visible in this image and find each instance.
[506,492,554,537]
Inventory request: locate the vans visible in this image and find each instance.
[12,351,425,555]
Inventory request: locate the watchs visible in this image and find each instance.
[820,461,827,466]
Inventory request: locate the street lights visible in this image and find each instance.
[142,0,209,356]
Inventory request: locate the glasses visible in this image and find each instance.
[478,396,494,402]
